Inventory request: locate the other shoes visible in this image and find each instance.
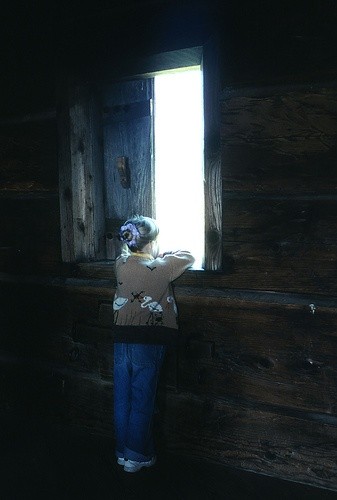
[117,456,161,473]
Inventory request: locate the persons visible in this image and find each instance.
[110,216,196,473]
